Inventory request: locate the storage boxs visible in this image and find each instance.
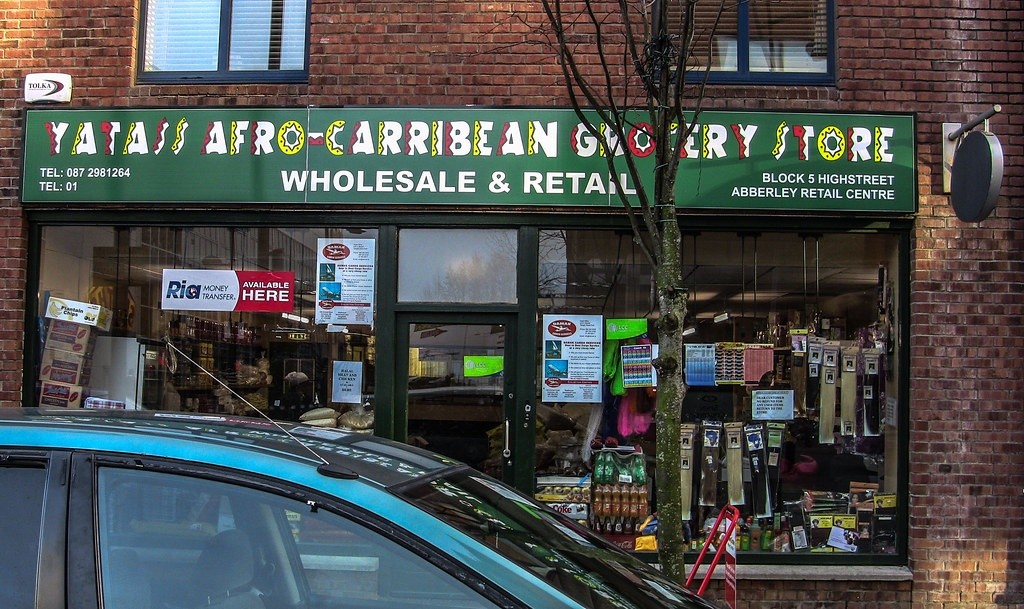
[45,296,114,331]
[38,319,98,408]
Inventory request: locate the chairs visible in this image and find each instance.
[107,547,152,609]
[189,528,264,609]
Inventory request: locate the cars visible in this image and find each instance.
[0,409,723,609]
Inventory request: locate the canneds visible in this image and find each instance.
[184,398,199,413]
[195,318,225,385]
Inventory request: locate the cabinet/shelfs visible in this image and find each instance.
[173,336,274,418]
[733,347,808,423]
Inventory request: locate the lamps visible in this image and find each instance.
[714,295,731,323]
[682,265,696,336]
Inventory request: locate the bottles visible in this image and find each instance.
[591,442,649,535]
[692,517,787,552]
